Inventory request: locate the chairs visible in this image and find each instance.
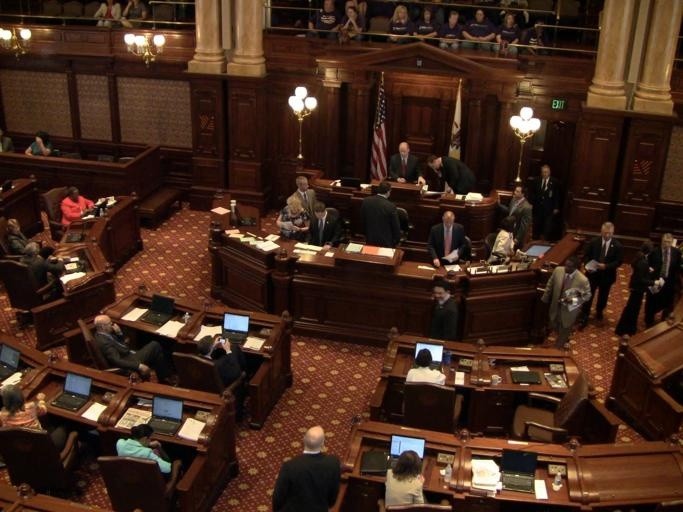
[62,2,83,20]
[510,374,589,443]
[36,186,81,244]
[96,446,185,512]
[458,238,476,264]
[0,260,57,327]
[173,349,248,423]
[367,17,390,43]
[84,1,101,20]
[394,208,411,249]
[153,0,174,21]
[49,143,64,156]
[377,486,454,512]
[324,209,343,241]
[0,425,79,499]
[390,380,465,433]
[40,1,62,19]
[484,233,499,261]
[74,318,151,379]
[0,216,44,257]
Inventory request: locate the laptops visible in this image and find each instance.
[147,395,184,437]
[386,433,426,472]
[140,293,175,327]
[413,341,444,371]
[221,310,250,344]
[50,371,93,413]
[65,222,87,243]
[83,199,109,217]
[510,371,542,385]
[500,446,539,493]
[0,344,22,382]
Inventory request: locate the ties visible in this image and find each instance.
[402,158,407,177]
[600,242,606,261]
[563,274,570,291]
[319,219,324,243]
[542,179,546,191]
[660,248,667,277]
[304,193,306,202]
[444,227,451,256]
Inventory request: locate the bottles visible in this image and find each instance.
[444,464,452,483]
[554,472,561,485]
[185,312,190,323]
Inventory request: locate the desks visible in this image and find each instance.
[324,412,682,511]
[0,174,38,233]
[356,328,619,439]
[62,287,300,429]
[0,126,162,202]
[203,187,586,344]
[609,292,682,443]
[0,334,240,510]
[296,162,518,226]
[20,191,144,351]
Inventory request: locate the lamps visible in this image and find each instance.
[0,26,32,63]
[284,86,317,159]
[507,106,541,182]
[121,32,166,65]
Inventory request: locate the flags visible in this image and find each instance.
[448,79,462,158]
[370,72,387,179]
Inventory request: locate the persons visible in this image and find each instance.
[59,186,95,224]
[540,255,591,348]
[427,211,465,267]
[342,6,367,40]
[428,280,457,340]
[385,450,425,505]
[307,202,342,250]
[6,218,28,253]
[462,9,496,49]
[116,425,184,480]
[388,5,415,44]
[94,0,120,28]
[523,20,550,56]
[0,130,15,153]
[20,242,64,289]
[25,131,53,157]
[120,0,150,30]
[0,386,69,456]
[271,426,339,512]
[440,11,465,50]
[390,142,426,185]
[359,182,401,246]
[415,6,441,45]
[644,233,683,328]
[196,336,241,386]
[578,222,625,328]
[427,154,476,195]
[489,217,516,264]
[93,314,178,376]
[527,164,560,241]
[309,0,339,37]
[496,13,521,58]
[496,185,533,263]
[615,240,660,336]
[406,348,446,385]
[276,196,310,239]
[500,0,530,22]
[293,175,317,219]
